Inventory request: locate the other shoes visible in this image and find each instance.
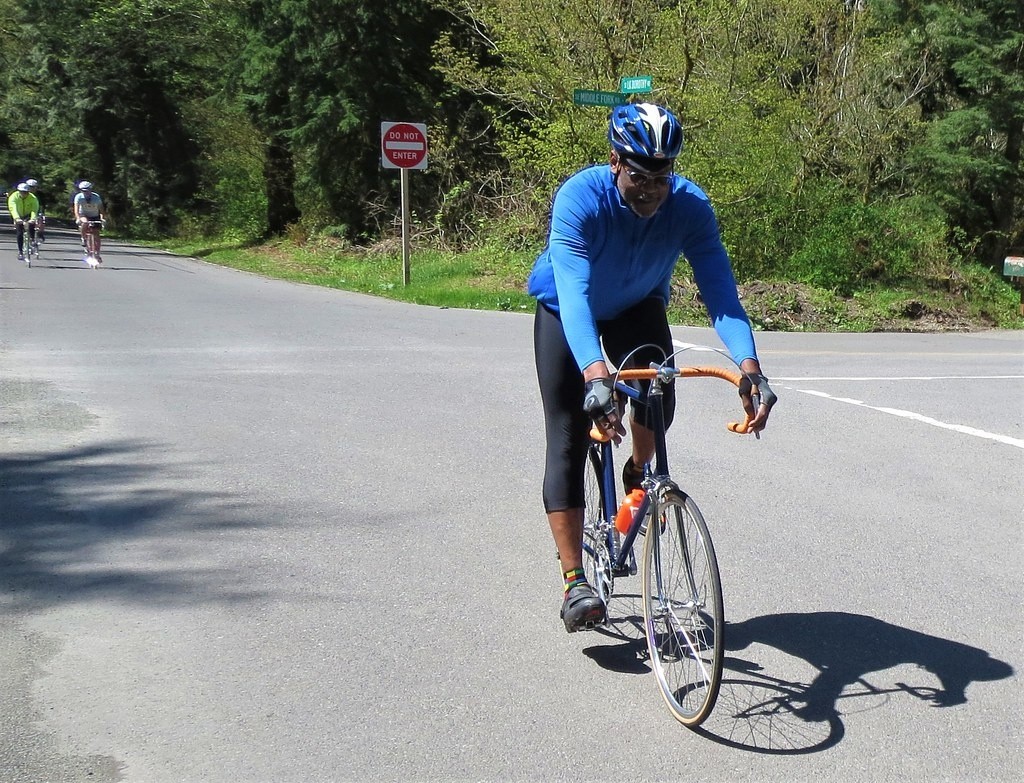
[17,250,24,260]
[82,239,86,247]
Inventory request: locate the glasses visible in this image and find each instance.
[622,165,675,186]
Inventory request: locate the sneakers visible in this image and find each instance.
[622,459,666,536]
[563,584,606,633]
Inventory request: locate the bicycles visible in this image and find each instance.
[80,220,106,267]
[15,220,35,269]
[30,219,44,260]
[581,366,756,729]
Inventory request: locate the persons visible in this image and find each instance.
[26,179,49,216]
[526,103,777,633]
[7,183,38,260]
[74,181,102,263]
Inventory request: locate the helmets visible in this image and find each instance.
[609,103,682,172]
[26,180,38,186]
[17,183,30,191]
[79,181,92,191]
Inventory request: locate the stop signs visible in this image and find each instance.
[381,121,428,170]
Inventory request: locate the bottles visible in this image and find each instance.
[616,490,645,534]
